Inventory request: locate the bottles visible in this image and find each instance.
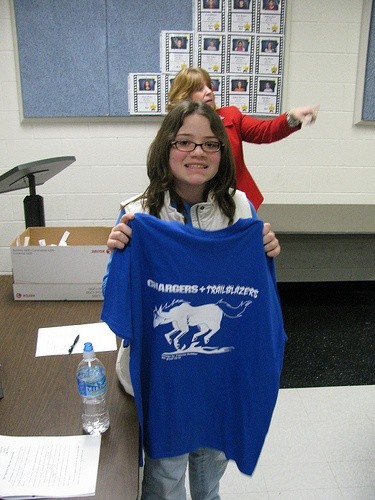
[76,342,111,435]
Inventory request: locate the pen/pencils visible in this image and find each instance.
[69,335,79,352]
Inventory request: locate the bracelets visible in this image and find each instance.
[286,111,299,128]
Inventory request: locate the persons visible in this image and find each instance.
[174,38,184,49]
[142,79,153,91]
[265,0,277,10]
[263,82,272,92]
[102,67,320,500]
[235,41,245,51]
[234,81,245,92]
[236,0,247,9]
[206,0,217,8]
[265,43,274,53]
[210,81,218,91]
[207,40,217,51]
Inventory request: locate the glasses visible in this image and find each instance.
[170,138,222,153]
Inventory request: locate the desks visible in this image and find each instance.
[256,204,375,280]
[0,274,143,500]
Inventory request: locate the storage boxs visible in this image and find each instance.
[10,226,112,302]
